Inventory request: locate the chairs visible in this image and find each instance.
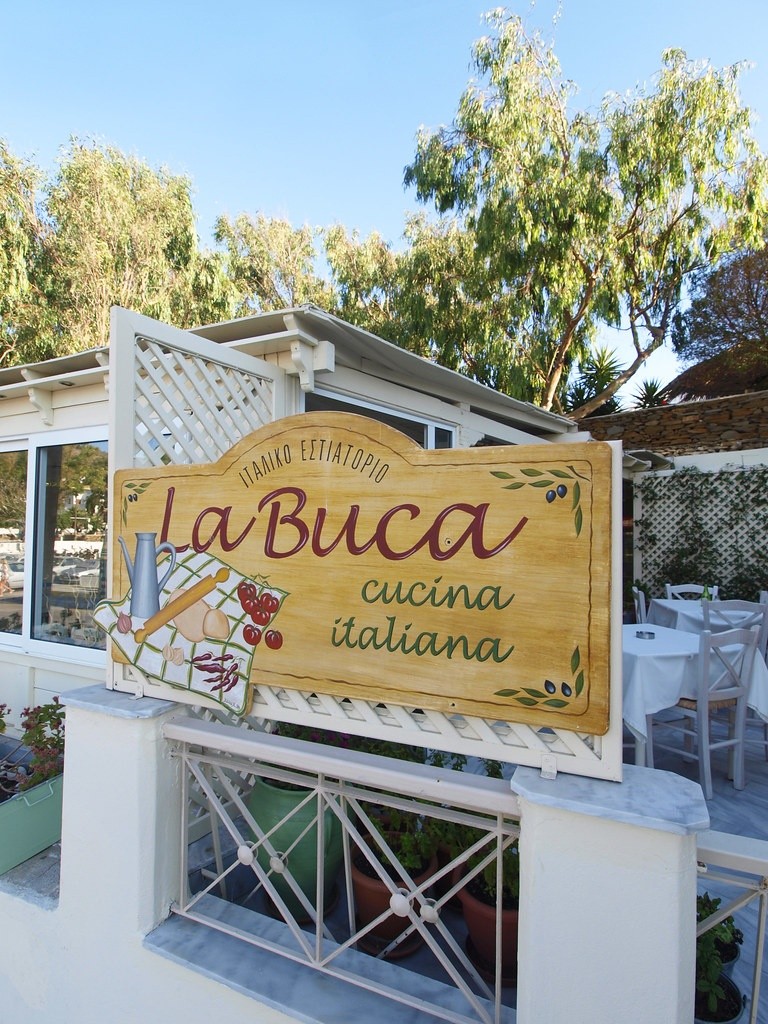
[647,625,762,799]
[666,583,719,601]
[631,585,648,624]
[701,597,768,667]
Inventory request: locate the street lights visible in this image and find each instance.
[72,490,78,541]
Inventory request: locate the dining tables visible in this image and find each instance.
[649,598,764,646]
[620,623,768,782]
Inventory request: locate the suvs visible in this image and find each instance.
[52,556,85,584]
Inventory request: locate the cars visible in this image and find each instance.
[5,563,25,589]
[60,560,99,583]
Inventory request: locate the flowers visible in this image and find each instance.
[264,718,352,749]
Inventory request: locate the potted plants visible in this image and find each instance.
[0,732,63,877]
[693,931,747,1024]
[449,820,520,986]
[697,890,745,976]
[349,801,439,959]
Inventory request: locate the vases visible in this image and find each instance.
[244,758,353,923]
